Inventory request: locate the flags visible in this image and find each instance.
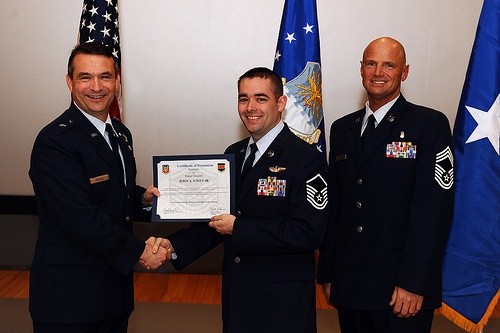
[433,0,500,333]
[72,0,123,127]
[263,0,330,284]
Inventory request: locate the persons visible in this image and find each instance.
[24,40,166,333]
[321,36,457,332]
[139,66,328,333]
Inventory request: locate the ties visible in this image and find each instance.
[362,114,376,141]
[105,123,118,163]
[242,143,258,175]
